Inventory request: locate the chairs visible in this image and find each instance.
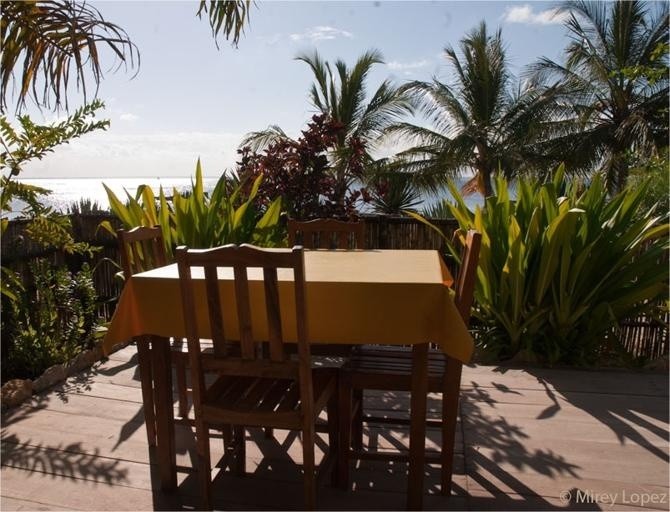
[338,228,483,498]
[116,223,238,447]
[287,217,368,250]
[174,242,347,512]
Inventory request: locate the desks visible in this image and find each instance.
[101,248,474,511]
